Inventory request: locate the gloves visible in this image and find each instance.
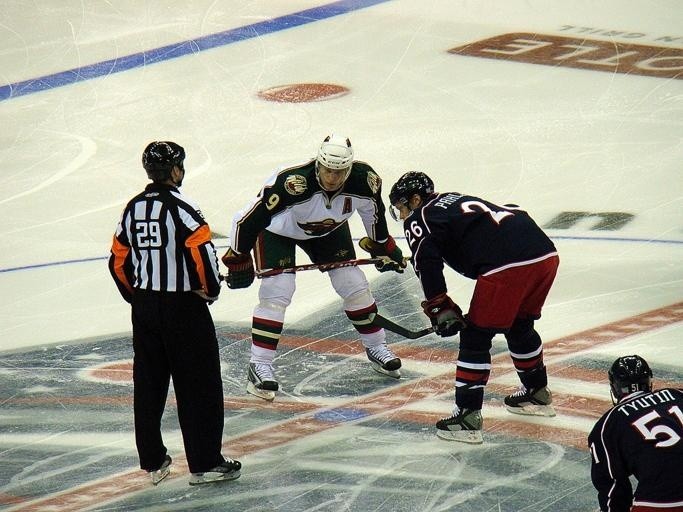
[421,295,468,337]
[222,246,254,289]
[359,237,407,273]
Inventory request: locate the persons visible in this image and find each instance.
[587,355,683,511]
[222,134,407,403]
[389,171,560,443]
[108,141,242,485]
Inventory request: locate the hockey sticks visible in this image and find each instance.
[368,314,469,339]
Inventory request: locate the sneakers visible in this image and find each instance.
[504,386,552,407]
[247,363,278,391]
[436,407,483,431]
[191,455,241,473]
[141,454,172,472]
[365,343,401,371]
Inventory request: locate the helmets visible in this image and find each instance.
[142,141,185,179]
[608,355,653,406]
[315,132,354,191]
[389,171,434,223]
